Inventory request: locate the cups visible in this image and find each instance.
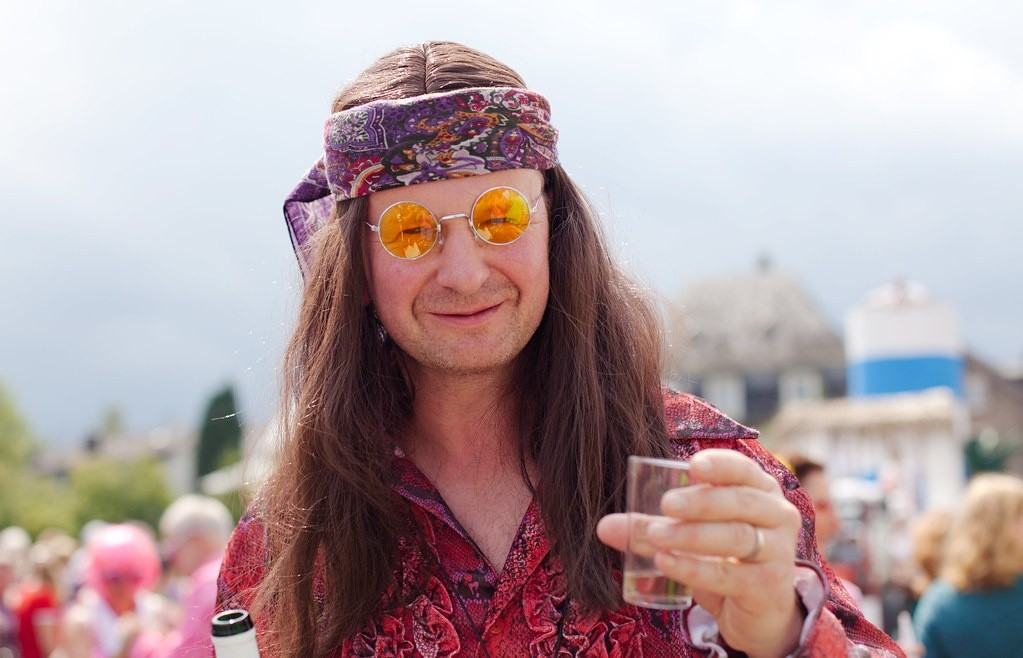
[623,454,700,611]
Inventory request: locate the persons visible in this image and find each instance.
[789,457,1023,658]
[211,41,904,658]
[0,492,235,658]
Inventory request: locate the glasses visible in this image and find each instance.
[365,186,544,261]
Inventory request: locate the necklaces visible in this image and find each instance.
[405,498,571,658]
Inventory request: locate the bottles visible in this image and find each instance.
[210,609,261,658]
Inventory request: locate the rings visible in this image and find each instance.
[740,528,765,562]
